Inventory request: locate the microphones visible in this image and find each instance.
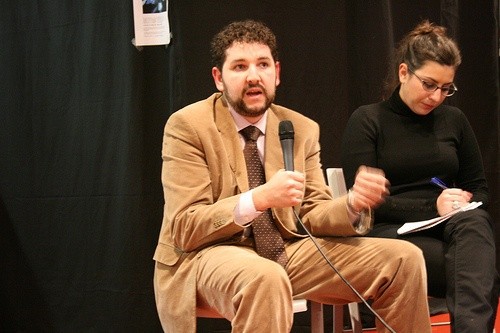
[279,120,295,172]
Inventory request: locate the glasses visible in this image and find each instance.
[408,66,457,97]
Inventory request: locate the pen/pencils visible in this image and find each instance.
[432,177,447,189]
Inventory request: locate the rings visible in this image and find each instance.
[453,200,460,209]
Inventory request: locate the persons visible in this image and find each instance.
[153,21,433,333]
[342,20,500,333]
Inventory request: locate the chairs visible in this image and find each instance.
[326,168,500,333]
[196,291,324,333]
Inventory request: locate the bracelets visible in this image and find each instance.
[347,187,365,216]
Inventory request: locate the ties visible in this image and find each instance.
[239,125,289,269]
